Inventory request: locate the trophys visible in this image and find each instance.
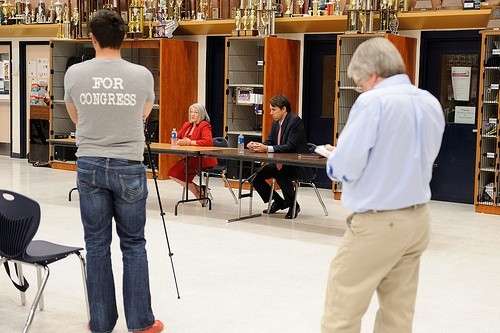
[0,0,399,38]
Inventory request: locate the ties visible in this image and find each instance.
[276,122,282,171]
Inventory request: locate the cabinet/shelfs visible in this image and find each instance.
[48,29,500,215]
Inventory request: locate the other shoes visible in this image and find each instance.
[285,203,301,219]
[133,320,164,333]
[201,185,211,207]
[263,199,288,214]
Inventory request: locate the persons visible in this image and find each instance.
[320,39,445,332]
[64,10,164,333]
[247,93,316,218]
[168,103,218,207]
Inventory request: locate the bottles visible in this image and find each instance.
[171,128,177,147]
[237,133,244,154]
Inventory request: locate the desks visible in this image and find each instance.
[47,138,238,215]
[199,150,328,223]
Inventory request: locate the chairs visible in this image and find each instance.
[181,137,238,208]
[266,142,329,219]
[0,188,91,333]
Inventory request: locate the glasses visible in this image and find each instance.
[353,78,364,94]
